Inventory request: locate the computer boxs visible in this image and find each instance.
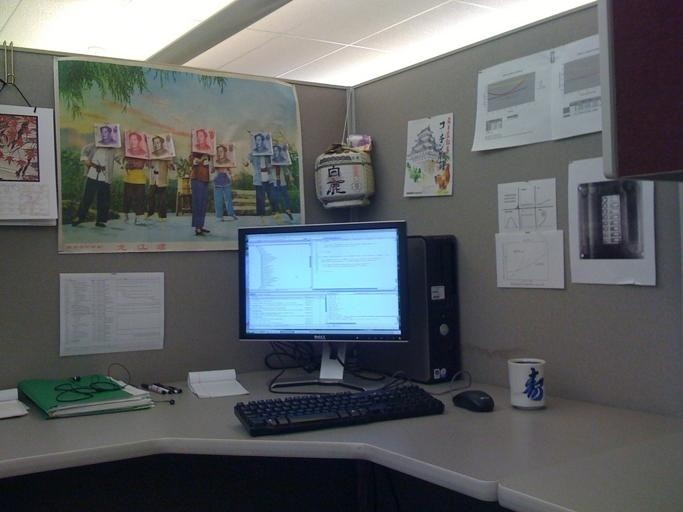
[360,236,462,383]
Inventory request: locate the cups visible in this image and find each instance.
[504,355,547,409]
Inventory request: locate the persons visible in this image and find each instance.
[75,120,294,237]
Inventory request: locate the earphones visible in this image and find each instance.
[74,376,78,381]
[170,400,175,404]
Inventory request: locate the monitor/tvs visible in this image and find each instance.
[238,220,407,392]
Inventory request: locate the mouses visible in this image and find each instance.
[453,390,494,412]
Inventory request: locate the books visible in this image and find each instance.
[18,375,156,419]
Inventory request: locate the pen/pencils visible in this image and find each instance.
[141,383,182,395]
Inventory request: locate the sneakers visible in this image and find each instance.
[195,226,211,238]
[257,209,294,226]
[124,212,169,226]
[71,216,107,228]
[217,213,239,221]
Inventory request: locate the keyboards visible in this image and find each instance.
[234,385,445,436]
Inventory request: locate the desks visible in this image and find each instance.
[493,425,681,512]
[0,375,681,512]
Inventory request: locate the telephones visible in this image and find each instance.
[578,178,642,259]
